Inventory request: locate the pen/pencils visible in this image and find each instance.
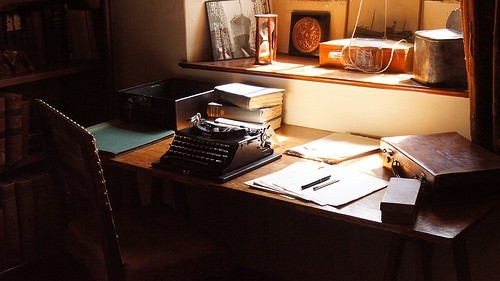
[301,175,331,189]
[313,179,340,191]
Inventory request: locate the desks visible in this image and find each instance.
[100,123,500,281]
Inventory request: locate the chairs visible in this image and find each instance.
[31,95,215,281]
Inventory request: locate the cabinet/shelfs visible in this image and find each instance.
[0,0,112,281]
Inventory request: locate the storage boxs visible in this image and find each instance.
[113,78,215,131]
[318,39,414,72]
[411,30,467,84]
[379,131,500,201]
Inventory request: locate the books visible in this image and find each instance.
[206,82,285,131]
